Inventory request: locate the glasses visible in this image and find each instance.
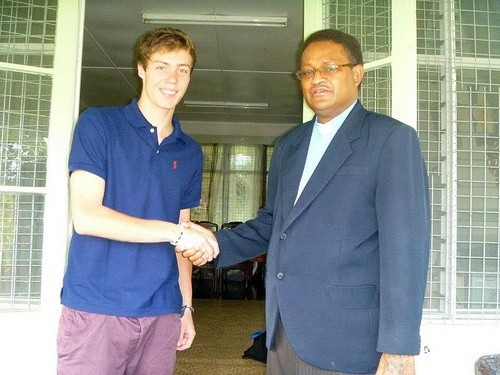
[295,62,356,80]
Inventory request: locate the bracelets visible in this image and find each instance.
[171,225,184,246]
[182,305,194,313]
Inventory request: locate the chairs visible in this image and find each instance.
[190,220,267,299]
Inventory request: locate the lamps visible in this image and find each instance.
[183,100,269,109]
[141,14,289,29]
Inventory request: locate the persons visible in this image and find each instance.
[54,27,220,375]
[174,29,431,375]
